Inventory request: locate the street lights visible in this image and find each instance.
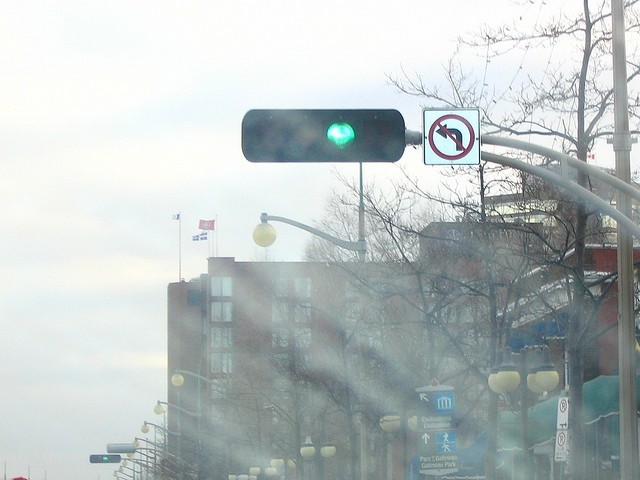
[488,345,559,480]
[265,454,296,480]
[171,369,211,394]
[253,163,366,476]
[133,438,166,460]
[154,401,202,449]
[300,437,336,480]
[379,410,418,480]
[141,421,181,458]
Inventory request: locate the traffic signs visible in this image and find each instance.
[415,380,459,475]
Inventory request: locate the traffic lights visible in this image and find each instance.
[242,110,405,162]
[90,455,121,463]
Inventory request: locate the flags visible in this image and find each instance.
[172,214,180,219]
[198,219,215,230]
[193,232,207,241]
